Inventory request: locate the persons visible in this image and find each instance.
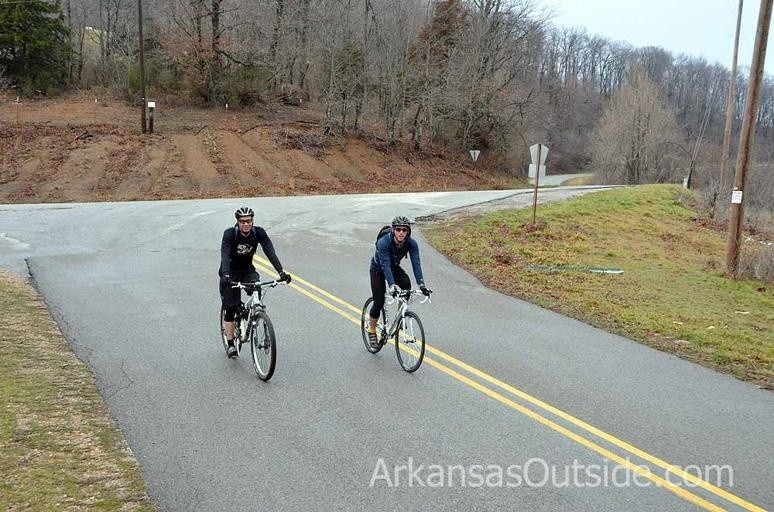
[366,215,429,348]
[217,207,292,359]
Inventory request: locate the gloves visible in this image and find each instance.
[420,286,432,296]
[222,273,231,285]
[280,272,291,284]
[389,285,401,297]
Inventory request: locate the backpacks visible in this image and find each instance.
[377,225,391,244]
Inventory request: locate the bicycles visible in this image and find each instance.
[219,278,289,382]
[360,287,433,374]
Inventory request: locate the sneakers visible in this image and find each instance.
[228,346,238,359]
[367,322,379,348]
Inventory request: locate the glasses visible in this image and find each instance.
[239,219,252,224]
[395,228,409,232]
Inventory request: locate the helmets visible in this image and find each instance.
[392,216,410,227]
[235,207,254,218]
